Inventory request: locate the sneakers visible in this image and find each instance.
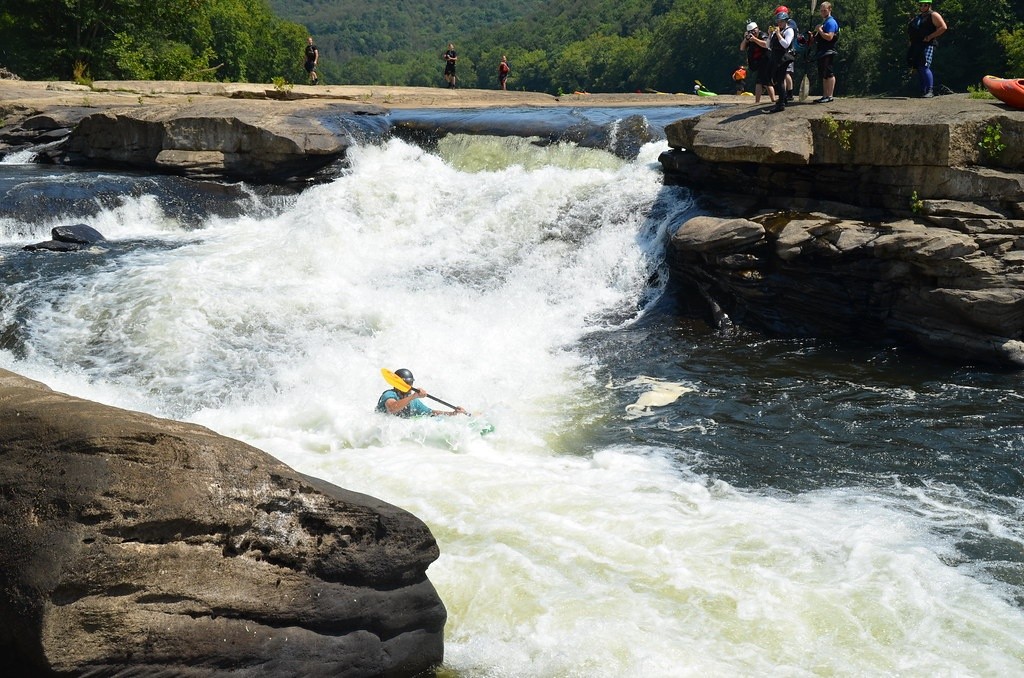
[813,97,833,103]
[447,82,456,89]
[762,95,794,114]
[311,78,319,85]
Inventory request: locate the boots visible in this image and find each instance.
[920,86,934,98]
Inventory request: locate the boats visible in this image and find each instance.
[980,74,1024,105]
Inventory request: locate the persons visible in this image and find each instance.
[771,5,799,99]
[304,37,319,85]
[442,43,457,90]
[811,2,840,102]
[739,22,776,104]
[496,55,510,90]
[765,12,794,114]
[907,0,947,98]
[376,368,464,419]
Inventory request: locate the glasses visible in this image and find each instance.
[918,4,930,7]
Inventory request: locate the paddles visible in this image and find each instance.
[380,368,471,417]
[695,78,709,92]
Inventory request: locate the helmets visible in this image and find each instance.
[774,6,788,15]
[394,368,415,382]
[919,0,932,3]
[776,12,789,21]
[747,22,758,32]
